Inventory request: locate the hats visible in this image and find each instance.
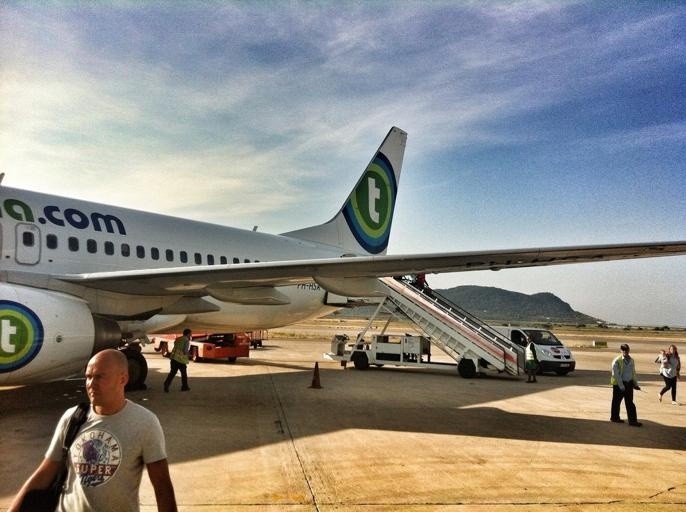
[621,344,628,350]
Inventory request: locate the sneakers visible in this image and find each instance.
[181,386,190,391]
[164,382,169,392]
[611,418,624,422]
[629,421,641,426]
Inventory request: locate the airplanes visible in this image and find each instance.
[0,125,686,387]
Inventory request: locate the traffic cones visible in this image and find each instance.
[307,362,322,388]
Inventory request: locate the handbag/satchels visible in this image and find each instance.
[18,484,60,512]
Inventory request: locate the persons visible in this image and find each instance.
[164,328,192,392]
[410,272,428,293]
[525,335,539,382]
[655,344,681,406]
[6,349,177,511]
[659,349,673,377]
[610,343,642,427]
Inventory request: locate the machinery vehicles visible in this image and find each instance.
[145,330,269,363]
[324,293,431,370]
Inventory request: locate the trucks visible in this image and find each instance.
[476,326,575,375]
